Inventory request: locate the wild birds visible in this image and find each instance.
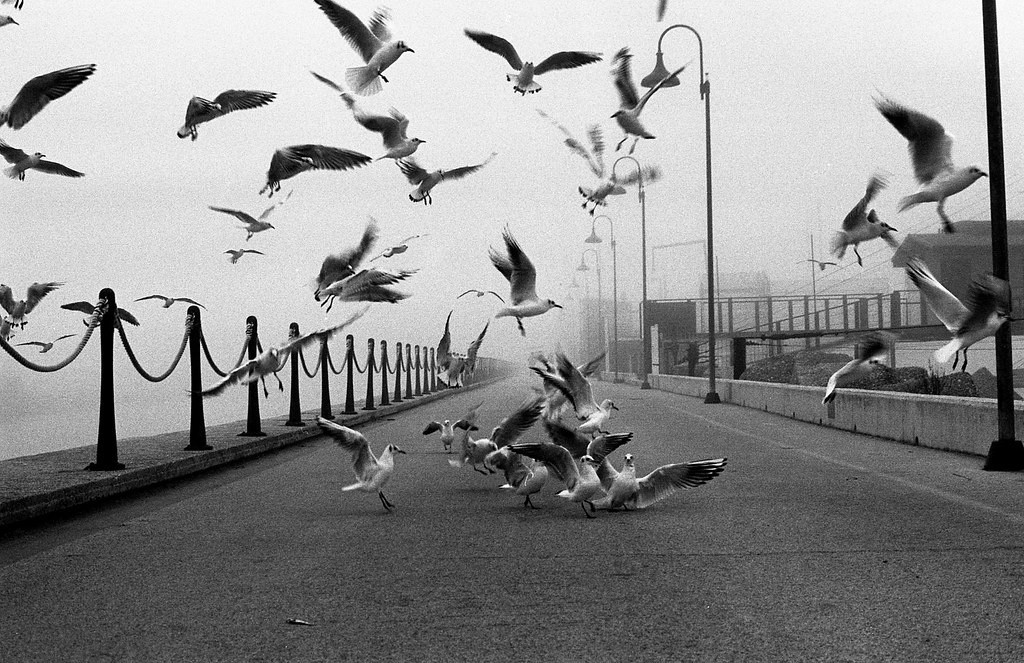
[533,354,607,419]
[831,180,899,265]
[563,1,692,218]
[869,89,986,236]
[315,415,407,513]
[906,256,1012,374]
[531,350,620,438]
[445,394,545,476]
[254,0,489,312]
[821,331,897,406]
[808,259,835,271]
[433,312,490,388]
[457,289,504,301]
[485,443,548,510]
[423,419,479,452]
[544,421,727,514]
[506,432,632,521]
[487,224,563,334]
[464,29,602,98]
[0,0,366,399]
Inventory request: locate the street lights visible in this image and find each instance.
[576,249,608,382]
[640,23,721,404]
[606,155,651,390]
[584,214,620,384]
